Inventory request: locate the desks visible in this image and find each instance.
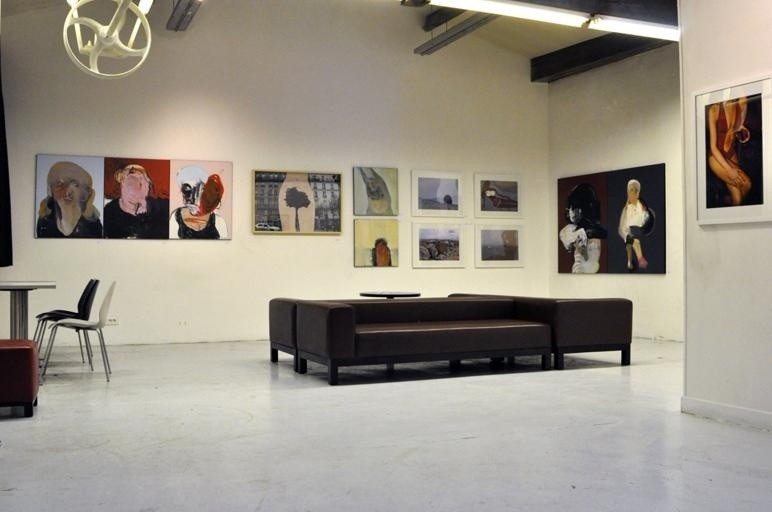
[0,281,56,341]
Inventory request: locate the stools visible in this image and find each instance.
[0,339,40,417]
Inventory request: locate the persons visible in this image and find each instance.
[704,97,752,205]
[277,172,317,233]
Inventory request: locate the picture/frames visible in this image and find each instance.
[695,78,772,225]
[411,222,466,269]
[475,224,525,268]
[474,174,523,219]
[412,170,464,218]
[252,169,343,235]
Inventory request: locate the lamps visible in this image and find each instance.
[430,0,590,28]
[414,11,500,56]
[166,1,203,32]
[588,14,680,43]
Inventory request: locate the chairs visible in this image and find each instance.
[41,281,117,381]
[38,279,99,370]
